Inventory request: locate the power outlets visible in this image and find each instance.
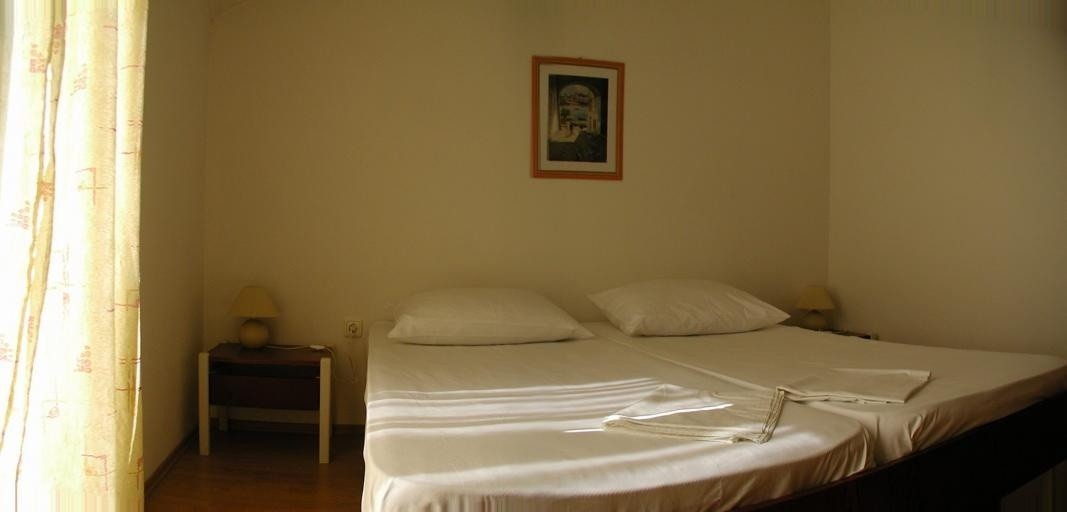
[343,319,361,339]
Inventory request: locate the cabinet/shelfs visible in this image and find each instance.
[194,340,335,466]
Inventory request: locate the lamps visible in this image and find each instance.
[794,283,835,329]
[225,283,282,349]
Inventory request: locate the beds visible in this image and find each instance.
[359,317,1066,512]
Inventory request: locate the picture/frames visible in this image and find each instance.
[528,53,626,184]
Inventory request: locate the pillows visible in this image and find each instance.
[586,277,791,339]
[387,287,596,346]
